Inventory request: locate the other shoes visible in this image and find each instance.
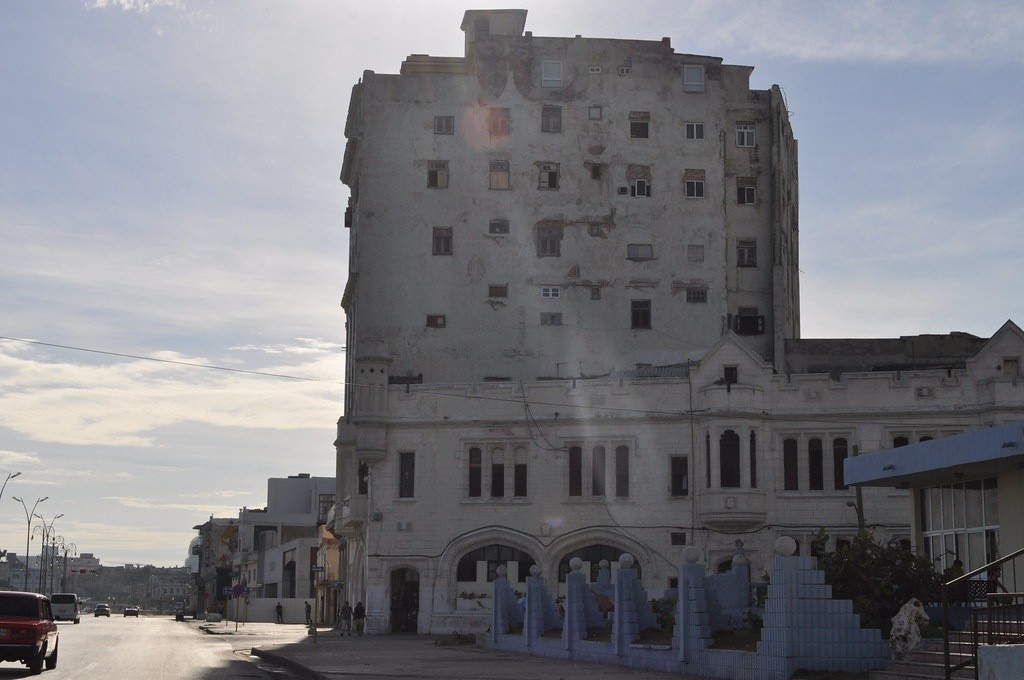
[341,633,343,635]
[348,633,351,636]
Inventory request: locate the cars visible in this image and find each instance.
[124,607,139,617]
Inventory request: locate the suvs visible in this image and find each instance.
[0,591,59,675]
[95,603,110,617]
[50,593,83,624]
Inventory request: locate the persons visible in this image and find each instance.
[218,603,224,614]
[989,567,1001,605]
[276,602,283,624]
[947,560,963,577]
[339,601,354,636]
[209,604,216,610]
[353,602,367,635]
[520,592,526,610]
[304,601,312,625]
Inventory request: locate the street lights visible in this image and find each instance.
[43,535,64,595]
[0,472,21,500]
[12,496,49,593]
[30,525,55,595]
[34,513,64,593]
[56,542,76,593]
